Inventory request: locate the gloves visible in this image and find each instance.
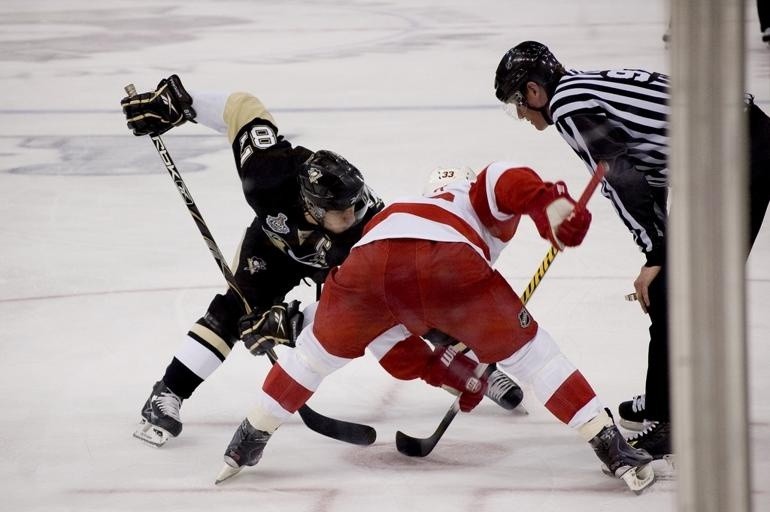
[529,180,592,252]
[429,344,488,412]
[238,300,301,355]
[121,74,197,136]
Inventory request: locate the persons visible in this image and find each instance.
[119,74,528,448]
[492,40,769,480]
[214,165,658,496]
[662,0,770,54]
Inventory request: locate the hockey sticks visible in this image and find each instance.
[126,84,377,445]
[396,160,605,456]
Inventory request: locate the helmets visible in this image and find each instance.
[495,40,560,118]
[300,150,370,229]
[427,165,475,191]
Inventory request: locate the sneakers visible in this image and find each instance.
[141,380,184,436]
[480,367,523,410]
[226,419,273,467]
[577,394,672,474]
